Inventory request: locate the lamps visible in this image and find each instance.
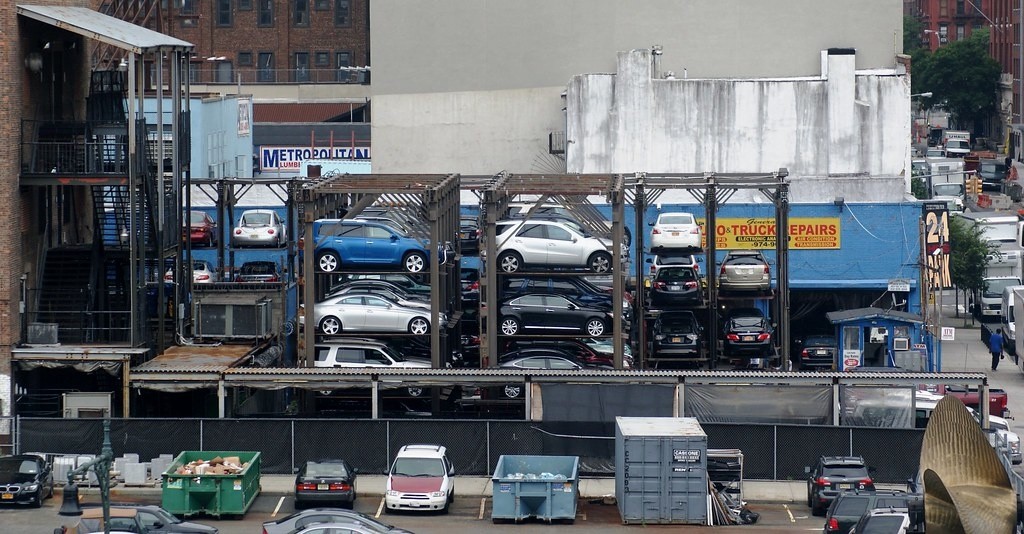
[118,58,128,71]
[911,92,933,98]
[834,197,844,213]
[120,229,129,241]
[778,168,788,177]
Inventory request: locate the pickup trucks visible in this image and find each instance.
[892,383,1015,421]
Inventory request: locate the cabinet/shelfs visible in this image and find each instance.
[707,449,744,509]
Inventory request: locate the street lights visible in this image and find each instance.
[58,452,110,534]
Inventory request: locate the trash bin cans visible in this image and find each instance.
[161,450,262,515]
[491,455,580,523]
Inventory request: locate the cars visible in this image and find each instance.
[262,508,415,534]
[0,455,54,509]
[294,458,358,510]
[649,267,705,306]
[150,203,634,400]
[723,309,778,356]
[102,505,219,534]
[648,213,705,253]
[794,328,835,372]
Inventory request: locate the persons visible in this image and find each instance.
[1003,156,1019,186]
[990,329,1004,371]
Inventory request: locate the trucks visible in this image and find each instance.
[912,112,1024,375]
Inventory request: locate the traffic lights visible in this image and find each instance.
[965,176,973,195]
[975,177,982,193]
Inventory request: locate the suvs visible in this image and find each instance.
[716,251,775,294]
[646,254,703,280]
[852,400,1022,464]
[383,442,455,515]
[54,507,170,534]
[649,312,706,359]
[804,455,876,515]
[303,337,452,396]
[848,509,910,534]
[823,488,908,534]
[845,390,1009,431]
